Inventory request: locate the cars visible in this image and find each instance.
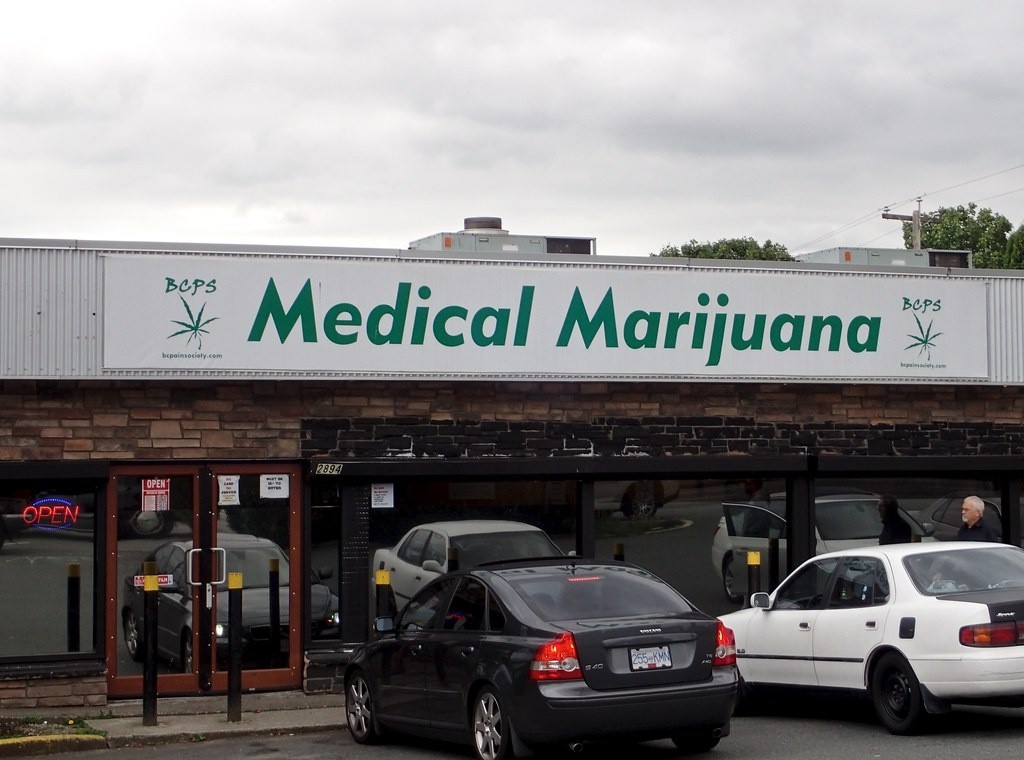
[370,516,593,612]
[908,494,1023,545]
[710,493,943,595]
[120,535,345,666]
[341,551,743,760]
[717,540,1024,736]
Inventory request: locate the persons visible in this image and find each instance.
[878,495,912,545]
[742,478,771,538]
[931,496,998,583]
